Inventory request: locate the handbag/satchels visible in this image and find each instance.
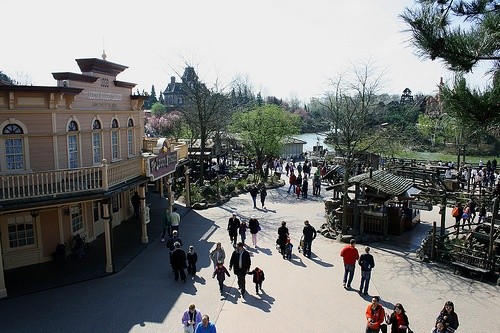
[405,326,413,333]
[256,220,261,231]
[381,324,387,333]
[183,261,187,269]
[166,214,171,226]
[184,324,193,333]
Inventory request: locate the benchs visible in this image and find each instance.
[452,252,493,282]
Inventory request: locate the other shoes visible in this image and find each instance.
[347,287,351,290]
[358,289,362,293]
[294,191,295,193]
[364,292,368,295]
[343,283,346,286]
[162,239,165,241]
[287,191,289,193]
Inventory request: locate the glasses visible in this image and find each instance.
[446,306,452,309]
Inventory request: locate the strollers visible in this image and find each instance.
[286,235,291,244]
[298,235,314,253]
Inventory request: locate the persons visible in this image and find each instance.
[182,304,216,333]
[379,158,384,170]
[340,239,374,296]
[131,191,141,218]
[365,296,409,333]
[400,159,417,177]
[216,146,252,167]
[347,199,358,214]
[429,158,500,191]
[352,160,372,177]
[455,198,487,230]
[250,148,337,209]
[209,242,230,292]
[229,242,265,296]
[303,221,317,258]
[161,207,198,283]
[432,301,459,333]
[227,212,261,248]
[278,221,293,261]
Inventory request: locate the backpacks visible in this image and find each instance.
[315,182,319,187]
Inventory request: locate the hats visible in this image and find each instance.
[251,215,255,218]
